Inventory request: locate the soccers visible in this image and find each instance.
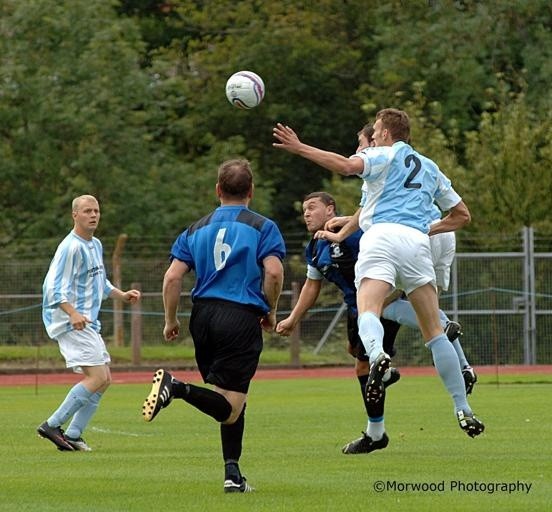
[226,71,265,110]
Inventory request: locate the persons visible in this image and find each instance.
[271,107,486,437]
[314,124,477,397]
[275,192,411,455]
[142,158,287,493]
[34,194,142,452]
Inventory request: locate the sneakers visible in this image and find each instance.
[461,364,477,397]
[364,347,401,406]
[223,474,256,494]
[63,434,93,452]
[36,419,77,451]
[430,321,463,366]
[457,409,485,438]
[341,431,389,454]
[141,368,180,422]
